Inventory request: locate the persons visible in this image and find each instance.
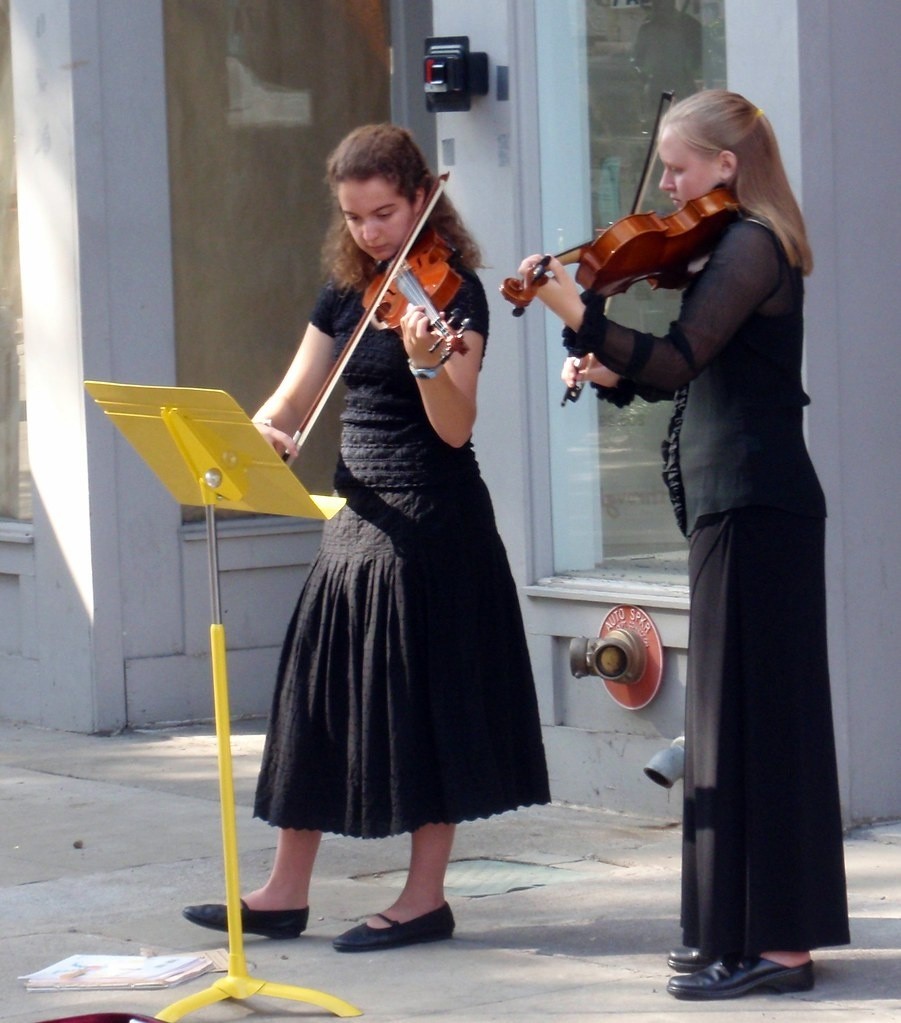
[519,89,851,1001]
[179,125,552,953]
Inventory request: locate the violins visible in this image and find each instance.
[362,226,473,366]
[498,185,745,318]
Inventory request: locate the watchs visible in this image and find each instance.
[408,358,444,379]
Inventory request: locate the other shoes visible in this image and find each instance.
[184,898,310,941]
[332,900,455,952]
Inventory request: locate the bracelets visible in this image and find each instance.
[250,418,272,428]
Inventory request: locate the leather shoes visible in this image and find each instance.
[667,947,722,973]
[666,952,815,1000]
